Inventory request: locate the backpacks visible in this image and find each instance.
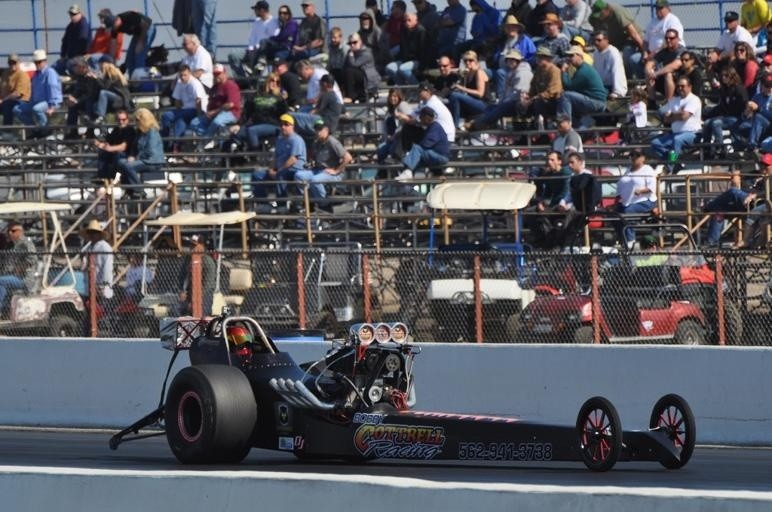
[221,137,249,167]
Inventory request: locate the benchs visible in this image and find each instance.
[0,40,770,304]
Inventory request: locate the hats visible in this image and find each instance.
[301,0,314,6]
[503,0,607,60]
[213,62,226,74]
[8,49,47,62]
[97,55,114,63]
[415,107,435,117]
[99,8,117,34]
[629,147,647,156]
[80,220,110,241]
[652,0,668,7]
[189,233,205,245]
[280,114,294,125]
[320,74,334,83]
[760,72,772,87]
[272,55,287,65]
[314,120,327,130]
[251,0,268,9]
[724,11,739,21]
[69,4,80,14]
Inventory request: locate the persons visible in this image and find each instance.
[226,325,255,368]
[0,1,771,259]
[0,220,38,320]
[79,217,117,329]
[172,233,222,322]
[312,302,347,337]
[114,249,159,303]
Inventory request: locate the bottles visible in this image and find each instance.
[667,148,677,162]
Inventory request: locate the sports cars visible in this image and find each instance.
[109,307,696,472]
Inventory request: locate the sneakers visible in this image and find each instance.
[394,169,413,180]
